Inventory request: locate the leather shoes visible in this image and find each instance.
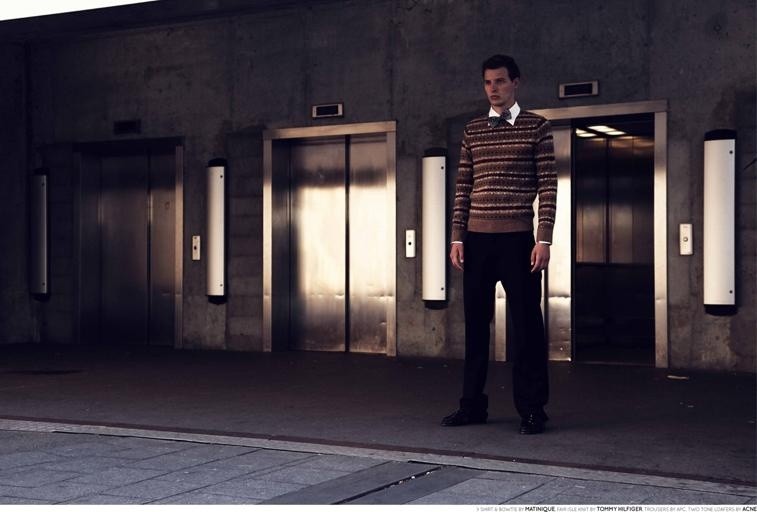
[441,406,489,429]
[519,408,550,435]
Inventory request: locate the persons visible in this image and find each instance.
[440,54,557,436]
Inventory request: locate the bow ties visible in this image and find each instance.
[487,107,514,128]
